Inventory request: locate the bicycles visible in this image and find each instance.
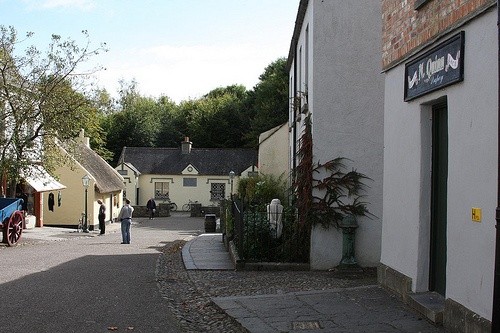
[162,199,178,212]
[77,212,90,234]
[182,200,198,212]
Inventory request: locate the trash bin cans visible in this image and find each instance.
[204,214,216,233]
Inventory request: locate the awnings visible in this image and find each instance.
[14,164,67,193]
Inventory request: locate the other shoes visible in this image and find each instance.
[97,233,104,236]
[121,242,127,244]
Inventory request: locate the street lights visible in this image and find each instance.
[228,171,235,200]
[81,173,91,233]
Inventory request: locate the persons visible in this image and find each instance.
[146,198,156,220]
[116,198,134,244]
[96,198,106,235]
